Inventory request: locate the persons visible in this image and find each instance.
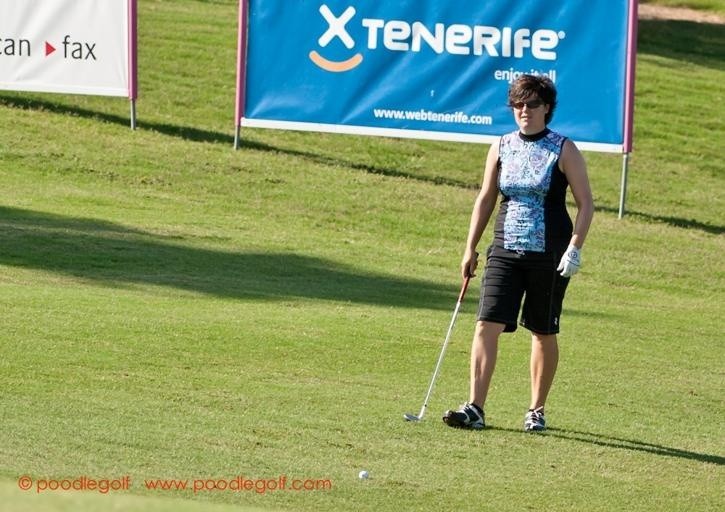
[444,72,595,431]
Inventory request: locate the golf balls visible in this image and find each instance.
[358,470,370,480]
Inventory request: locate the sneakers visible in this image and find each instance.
[524,408,546,432]
[442,402,486,429]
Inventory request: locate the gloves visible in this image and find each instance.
[556,243,584,278]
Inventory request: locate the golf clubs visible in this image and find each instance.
[402,252,479,422]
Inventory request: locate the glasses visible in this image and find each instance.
[513,100,543,110]
